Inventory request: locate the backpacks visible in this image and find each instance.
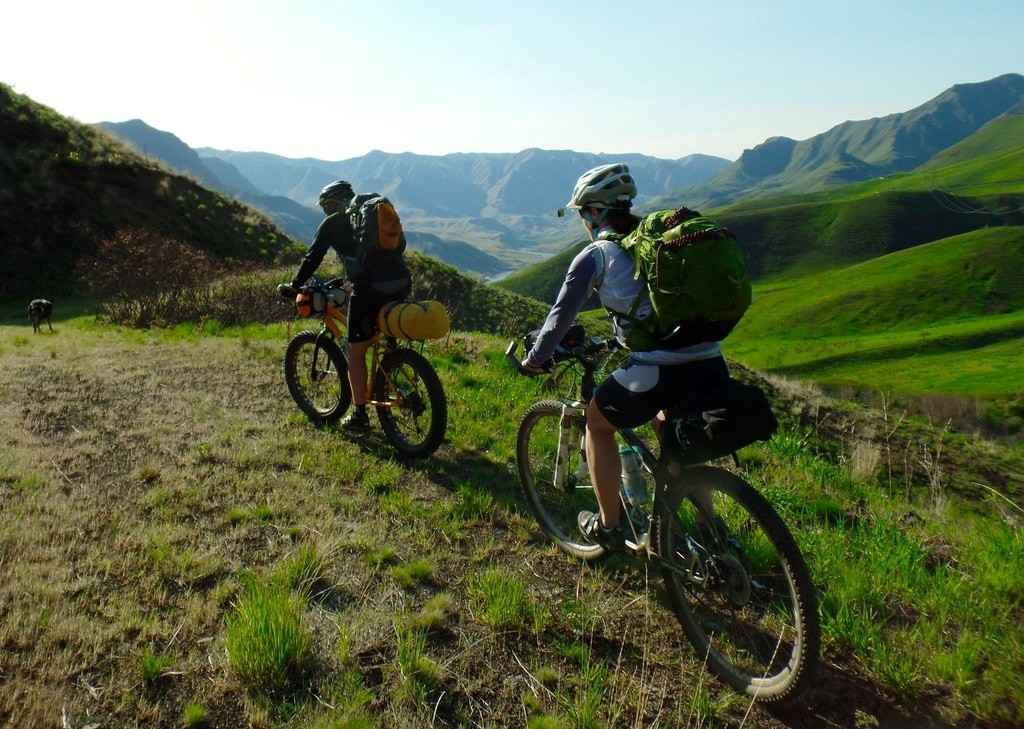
[346,192,407,257]
[597,206,753,351]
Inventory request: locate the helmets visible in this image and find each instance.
[315,180,355,206]
[565,163,638,210]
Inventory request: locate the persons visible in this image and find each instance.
[516,163,739,558]
[281,181,412,434]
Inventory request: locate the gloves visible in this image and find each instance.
[278,284,297,293]
[521,358,545,374]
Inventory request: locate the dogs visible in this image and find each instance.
[28,300,54,333]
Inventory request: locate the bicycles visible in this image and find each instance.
[505,331,822,706]
[283,277,449,456]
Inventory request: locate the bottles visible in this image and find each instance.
[338,335,351,357]
[618,444,649,506]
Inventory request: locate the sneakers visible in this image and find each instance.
[578,510,626,555]
[697,516,729,543]
[341,410,370,432]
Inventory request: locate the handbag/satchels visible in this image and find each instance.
[660,376,780,464]
[379,301,449,339]
